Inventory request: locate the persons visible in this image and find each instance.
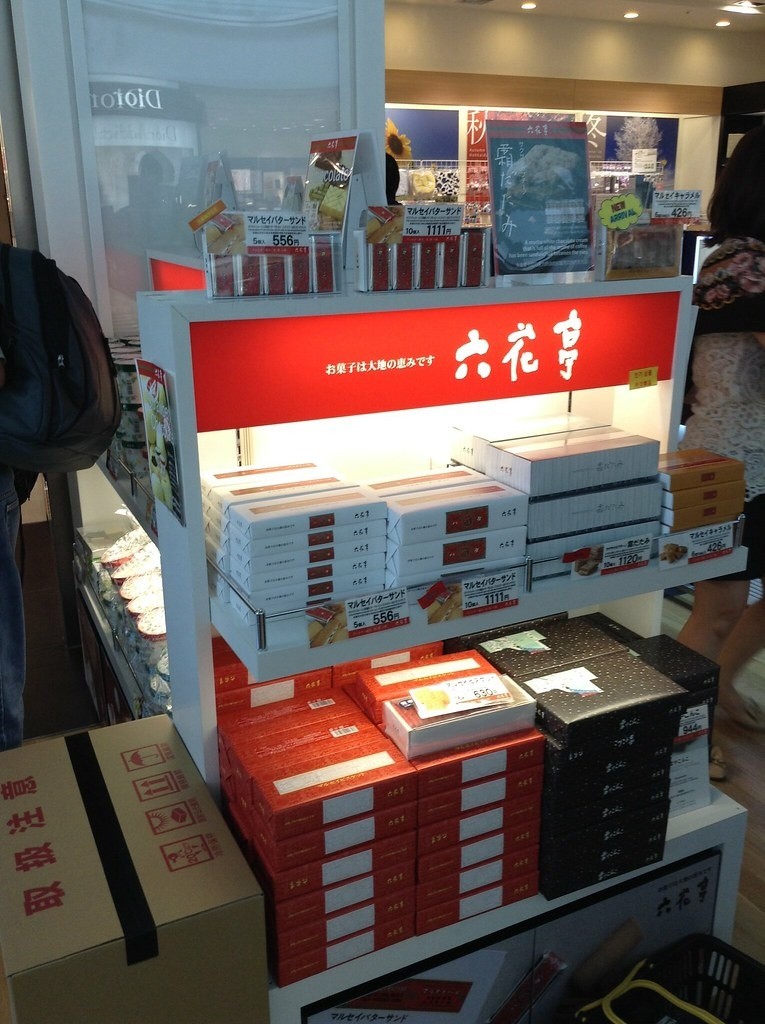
[677,125,765,778]
[384,153,403,206]
[0,236,27,755]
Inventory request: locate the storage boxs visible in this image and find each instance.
[203,411,745,617]
[0,714,271,1024]
[212,611,722,989]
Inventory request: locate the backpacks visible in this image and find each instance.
[0,244,123,474]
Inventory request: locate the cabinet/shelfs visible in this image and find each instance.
[136,274,749,1024]
[71,443,156,727]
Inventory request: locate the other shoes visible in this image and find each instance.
[723,697,765,731]
[710,746,728,779]
